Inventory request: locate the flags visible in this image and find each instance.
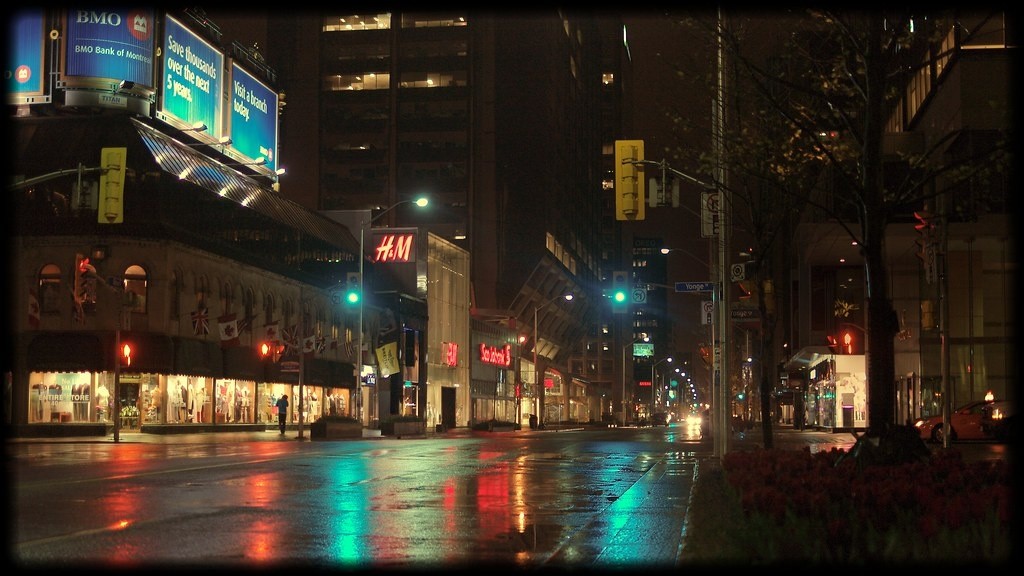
[189,306,369,362]
[446,341,459,368]
[27,284,85,328]
[374,341,400,378]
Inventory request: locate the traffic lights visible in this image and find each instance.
[827,334,838,347]
[736,393,744,400]
[842,329,854,345]
[121,341,133,368]
[259,342,271,358]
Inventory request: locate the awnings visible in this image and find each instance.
[124,117,370,262]
[114,329,283,383]
[22,332,107,375]
[549,365,595,391]
[303,359,358,392]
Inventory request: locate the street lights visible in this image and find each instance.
[80,263,123,441]
[622,337,650,427]
[534,293,573,431]
[651,357,673,405]
[662,368,680,399]
[660,247,722,459]
[357,198,428,422]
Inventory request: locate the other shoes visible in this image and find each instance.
[279,433,287,438]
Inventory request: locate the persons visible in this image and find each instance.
[276,395,288,436]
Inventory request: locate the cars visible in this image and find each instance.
[913,398,1008,445]
[651,412,671,428]
